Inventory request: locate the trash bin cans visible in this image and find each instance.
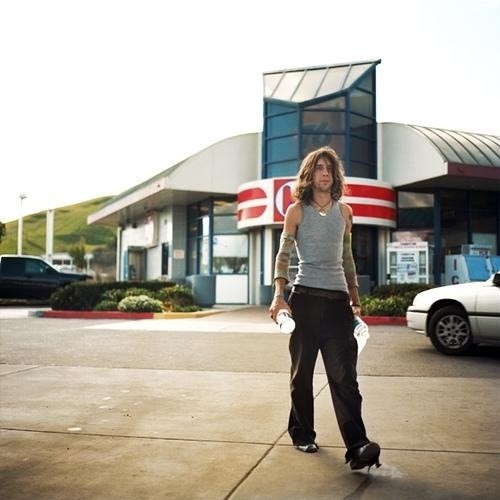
[259,285,272,306]
[191,274,215,308]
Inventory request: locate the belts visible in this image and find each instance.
[290,285,348,301]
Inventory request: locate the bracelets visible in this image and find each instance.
[272,293,285,299]
[351,302,361,308]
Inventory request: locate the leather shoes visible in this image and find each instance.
[349,441,381,469]
[296,443,318,453]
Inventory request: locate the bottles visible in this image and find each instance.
[353,314,371,340]
[276,309,296,335]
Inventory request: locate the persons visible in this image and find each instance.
[267,145,384,476]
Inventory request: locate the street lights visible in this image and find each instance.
[17,193,28,256]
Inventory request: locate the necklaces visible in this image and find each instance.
[310,198,334,219]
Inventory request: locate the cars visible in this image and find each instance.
[404,269,500,356]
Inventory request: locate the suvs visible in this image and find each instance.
[0,255,97,303]
